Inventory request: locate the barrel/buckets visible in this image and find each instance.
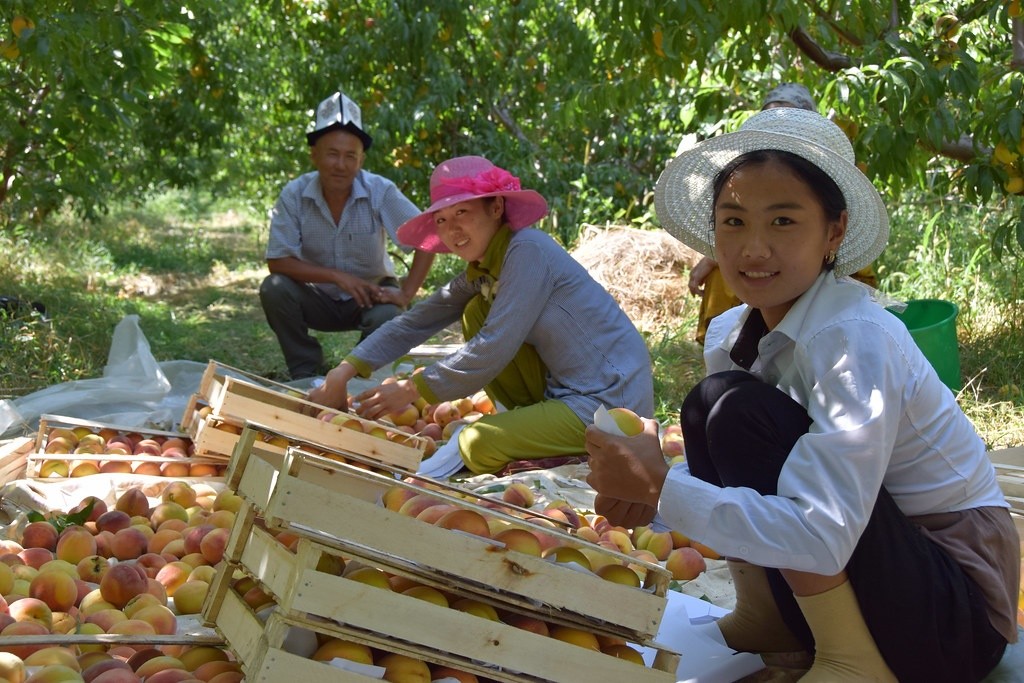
[884,299,962,399]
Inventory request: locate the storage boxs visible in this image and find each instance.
[1,357,682,682]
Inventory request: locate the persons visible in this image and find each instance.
[649,106,1022,682]
[259,92,438,382]
[688,86,879,351]
[305,155,655,478]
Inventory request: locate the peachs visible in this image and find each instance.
[0,0,1024,195]
[0,367,721,682]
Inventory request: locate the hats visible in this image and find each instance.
[306,90,374,152]
[653,110,891,279]
[761,83,816,112]
[397,155,547,253]
